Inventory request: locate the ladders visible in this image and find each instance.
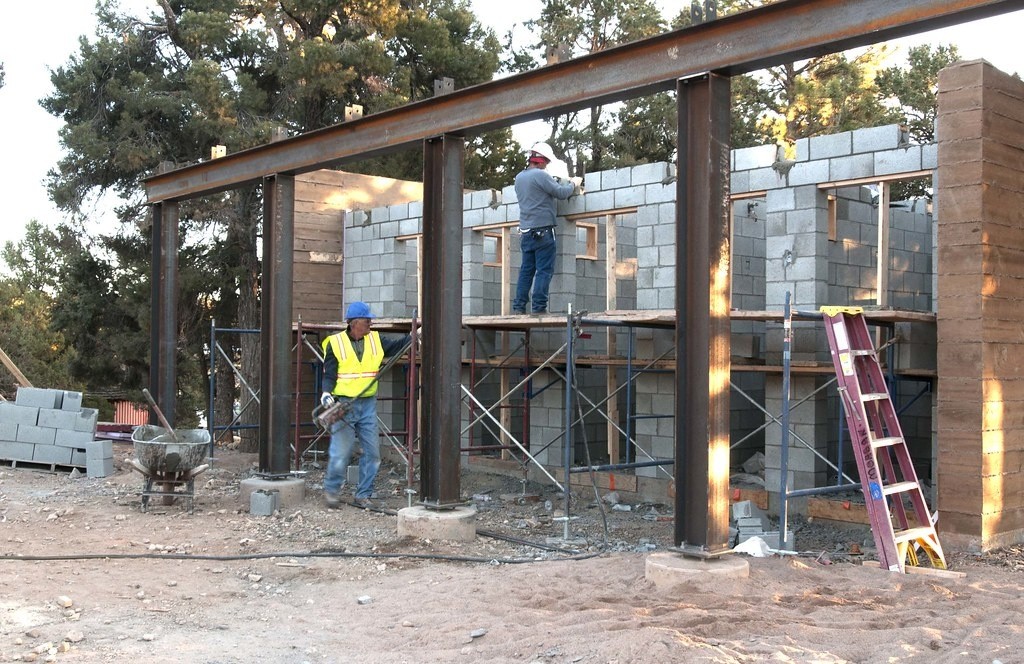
[818,304,950,575]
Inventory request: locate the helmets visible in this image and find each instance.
[345,302,376,318]
[530,142,554,161]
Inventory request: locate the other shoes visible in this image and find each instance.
[324,491,339,507]
[532,310,549,316]
[354,498,375,508]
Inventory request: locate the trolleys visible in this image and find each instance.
[126,422,210,514]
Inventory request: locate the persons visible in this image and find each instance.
[512,142,584,319]
[319,302,421,511]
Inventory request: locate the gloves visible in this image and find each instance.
[417,327,422,339]
[320,392,334,409]
[571,176,583,187]
